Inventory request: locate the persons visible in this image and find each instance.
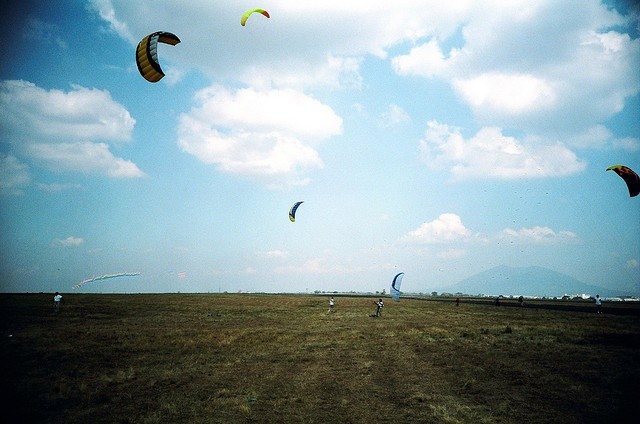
[496,297,500,306]
[520,299,526,310]
[49,291,63,315]
[327,297,336,314]
[455,298,459,305]
[594,294,603,314]
[374,299,384,317]
